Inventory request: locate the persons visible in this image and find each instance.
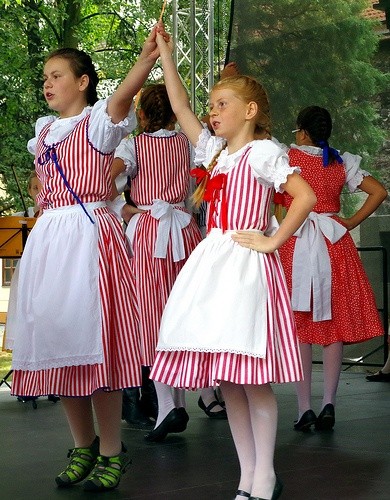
[9,170,41,218]
[110,83,204,446]
[148,20,317,500]
[273,106,388,430]
[3,21,170,491]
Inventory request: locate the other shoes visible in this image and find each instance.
[365,368,390,381]
[293,409,317,431]
[315,403,335,431]
[214,389,225,409]
[54,436,100,486]
[143,407,180,444]
[249,472,282,500]
[82,442,132,492]
[197,392,226,419]
[174,407,189,433]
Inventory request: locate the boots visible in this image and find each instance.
[121,386,148,425]
[141,365,159,421]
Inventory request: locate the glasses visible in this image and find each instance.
[291,128,303,135]
